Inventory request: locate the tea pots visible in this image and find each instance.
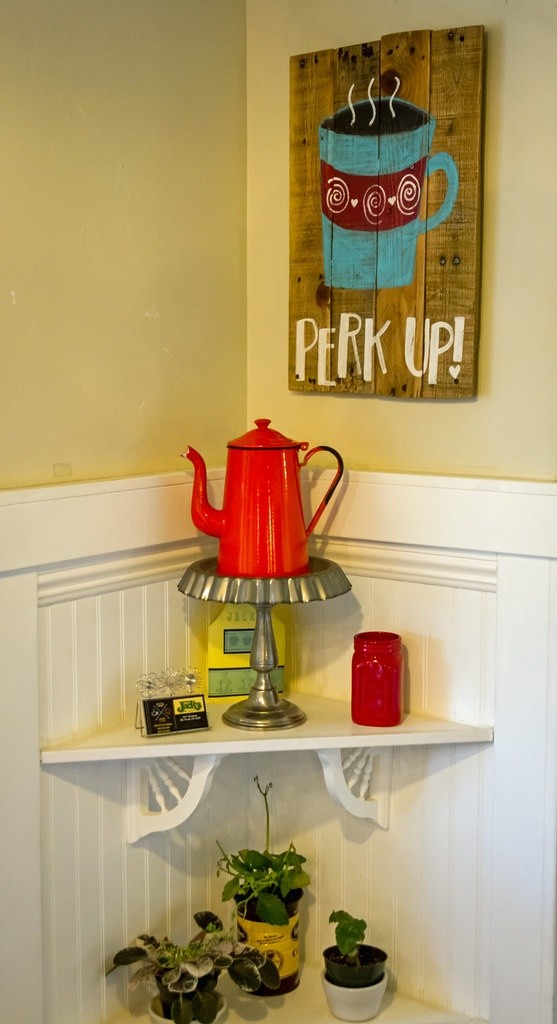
[179,419,345,582]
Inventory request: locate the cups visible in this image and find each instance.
[316,97,458,289]
[348,630,403,727]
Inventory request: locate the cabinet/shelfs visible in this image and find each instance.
[40,692,495,1024]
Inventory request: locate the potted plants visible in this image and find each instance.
[104,911,281,1024]
[321,909,388,1021]
[215,776,311,997]
